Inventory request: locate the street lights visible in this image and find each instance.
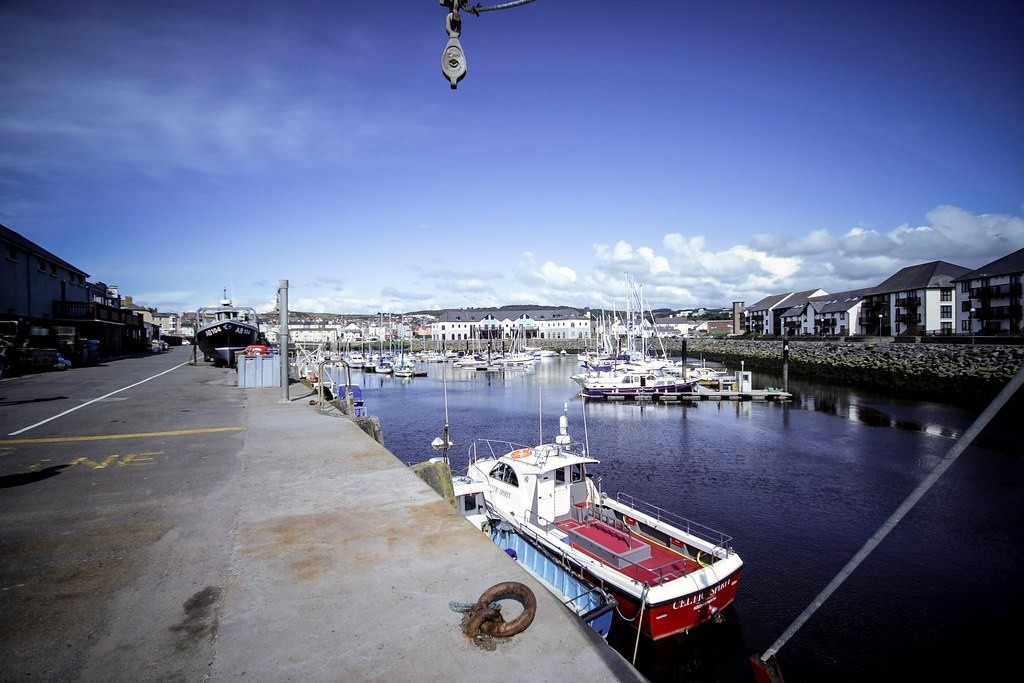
[969,308,976,345]
[820,318,824,341]
[879,313,884,346]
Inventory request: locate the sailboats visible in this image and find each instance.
[568,271,794,403]
[291,307,558,381]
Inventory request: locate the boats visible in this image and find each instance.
[194,287,260,367]
[464,385,744,645]
[336,383,368,418]
